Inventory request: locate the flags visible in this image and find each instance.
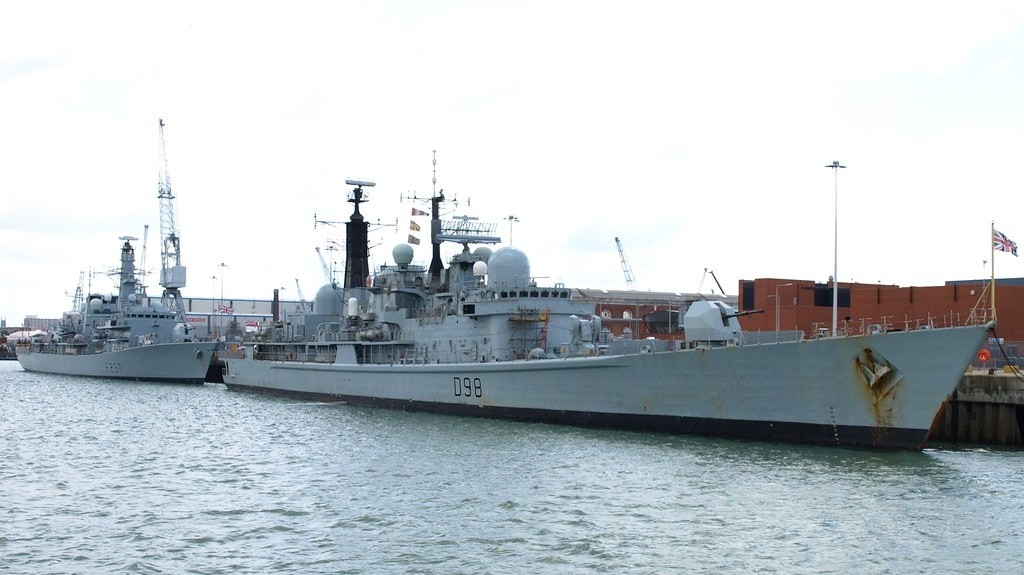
[992,228,1018,258]
[218,304,235,315]
[409,220,420,231]
[408,234,420,245]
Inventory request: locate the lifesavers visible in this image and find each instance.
[288,352,296,360]
[867,324,882,335]
[979,350,990,362]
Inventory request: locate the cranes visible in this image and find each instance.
[615,237,638,291]
[154,117,188,321]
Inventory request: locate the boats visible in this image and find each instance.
[217,146,998,452]
[14,234,221,384]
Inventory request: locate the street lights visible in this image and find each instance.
[280,287,286,320]
[217,263,229,342]
[503,215,520,247]
[775,282,793,330]
[825,160,848,337]
[211,276,218,336]
[768,294,780,330]
